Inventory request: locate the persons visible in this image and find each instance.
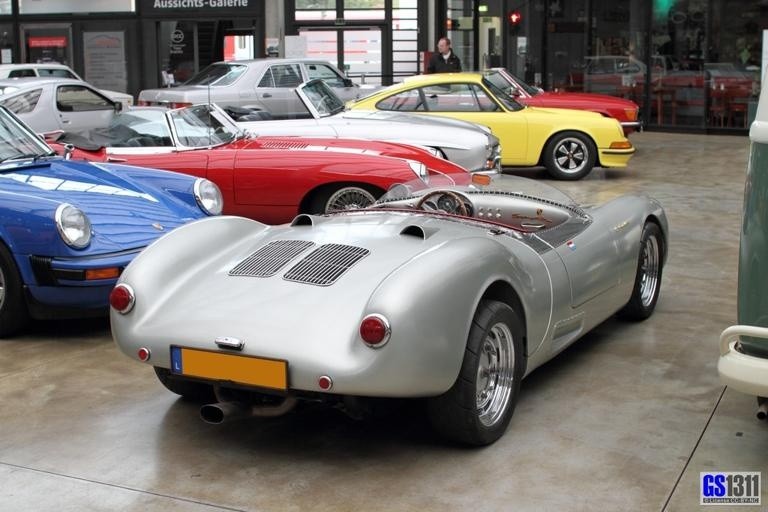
[543,13,760,91]
[268,47,280,59]
[425,38,463,90]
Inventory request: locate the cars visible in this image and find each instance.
[716,55,768,421]
[534,52,761,125]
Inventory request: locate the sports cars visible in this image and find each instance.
[0,57,670,449]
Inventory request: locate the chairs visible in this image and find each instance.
[279,75,299,87]
[568,65,761,130]
[127,136,157,146]
